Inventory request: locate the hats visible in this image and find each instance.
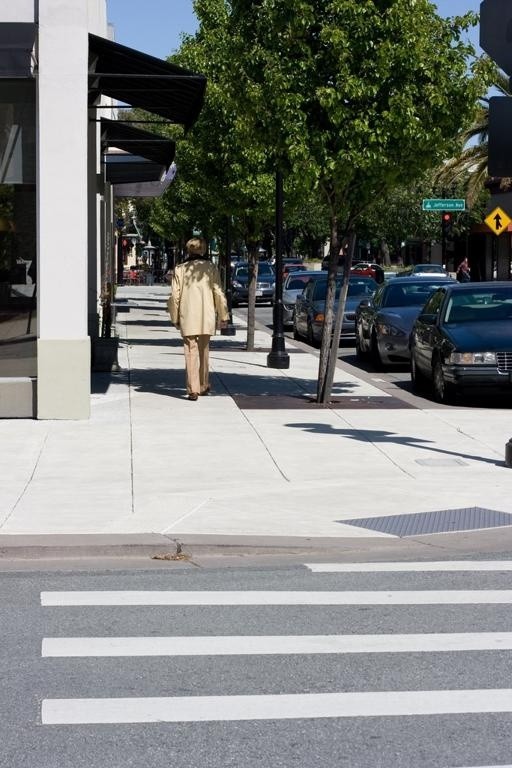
[185,238,207,254]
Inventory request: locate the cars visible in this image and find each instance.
[11,257,36,298]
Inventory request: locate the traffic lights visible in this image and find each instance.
[442,212,453,235]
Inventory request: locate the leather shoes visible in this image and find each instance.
[188,385,211,400]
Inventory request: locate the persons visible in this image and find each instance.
[168,237,230,401]
[456,256,471,283]
